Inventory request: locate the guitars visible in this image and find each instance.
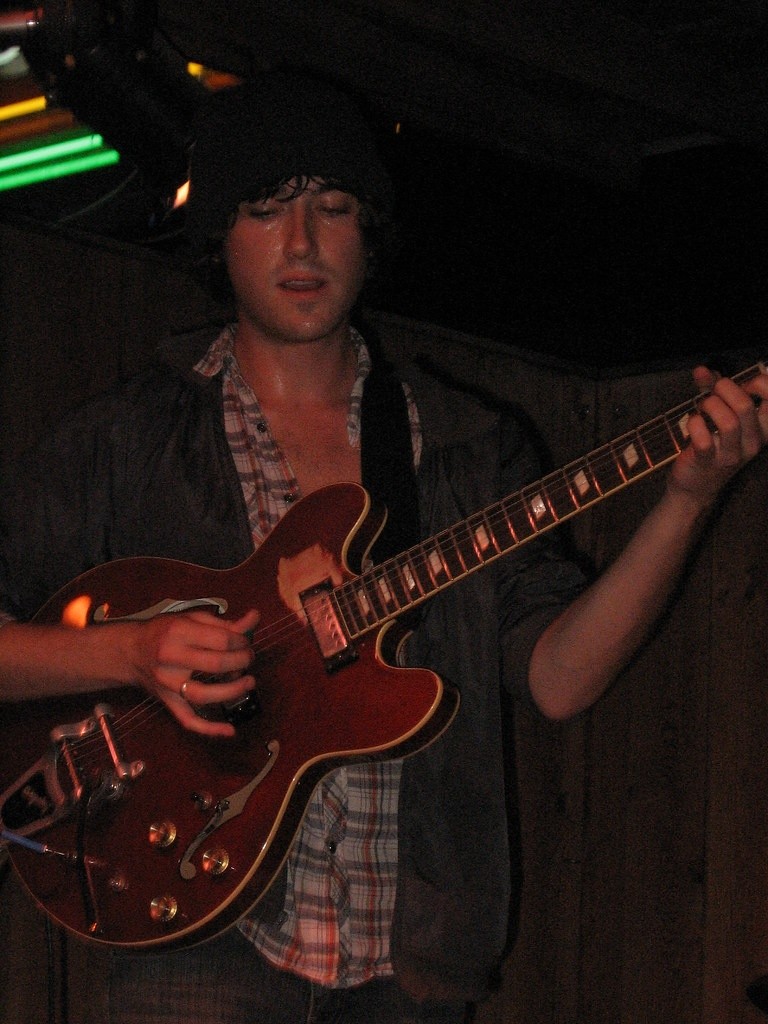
[0,360,768,958]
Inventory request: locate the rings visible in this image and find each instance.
[177,679,193,699]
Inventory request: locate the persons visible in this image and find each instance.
[0,81,767,1022]
